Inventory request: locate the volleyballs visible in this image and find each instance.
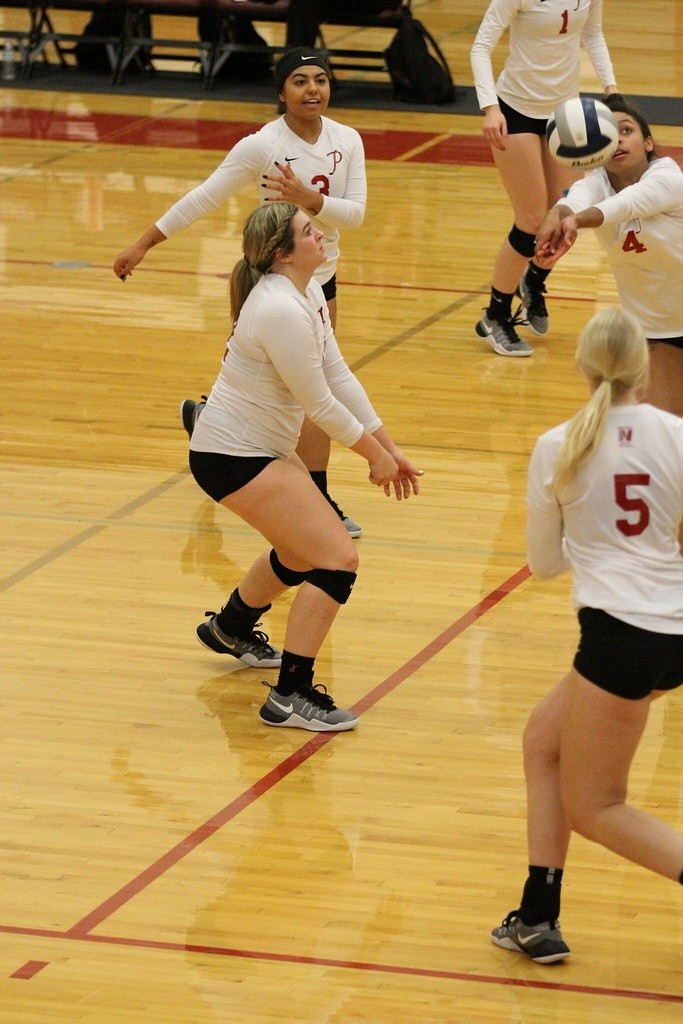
[545,96,620,170]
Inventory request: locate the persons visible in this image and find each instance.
[468,1,618,358]
[490,303,683,966]
[531,94,683,418]
[113,49,365,537]
[188,204,427,732]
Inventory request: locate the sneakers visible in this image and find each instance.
[519,270,549,338]
[180,394,208,442]
[323,492,363,537]
[474,307,535,363]
[488,910,571,966]
[193,610,283,670]
[257,679,362,733]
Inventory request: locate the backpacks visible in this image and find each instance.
[197,12,274,86]
[382,7,456,106]
[74,8,152,75]
